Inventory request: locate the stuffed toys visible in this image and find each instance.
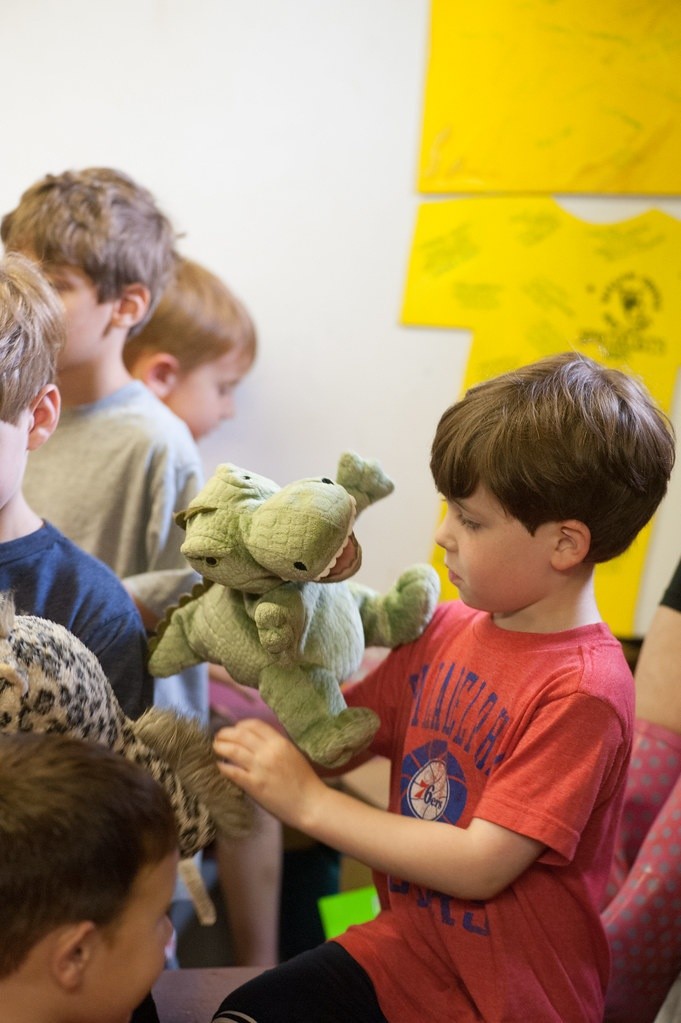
[0,596,262,856]
[148,452,440,768]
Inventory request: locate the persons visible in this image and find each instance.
[0,734,180,1023]
[123,257,283,968]
[0,165,204,721]
[0,253,153,722]
[603,559,681,1022]
[208,352,674,1023]
